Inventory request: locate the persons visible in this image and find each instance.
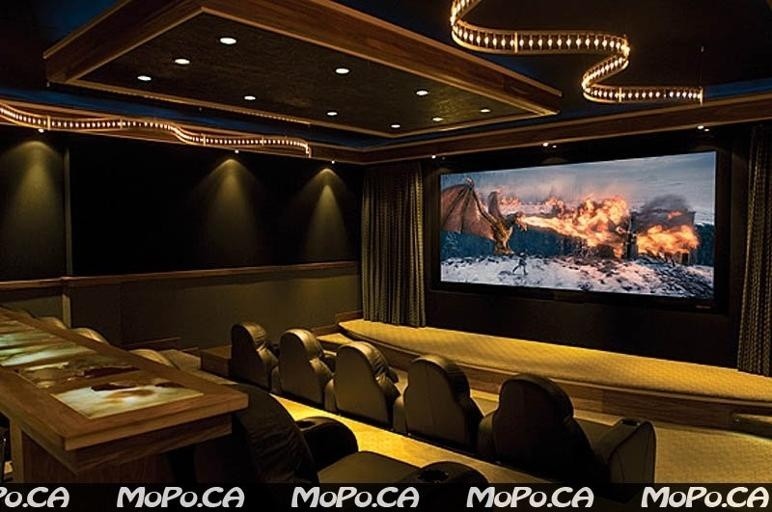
[512,252,529,276]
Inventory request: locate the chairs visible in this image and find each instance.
[0,297,657,483]
